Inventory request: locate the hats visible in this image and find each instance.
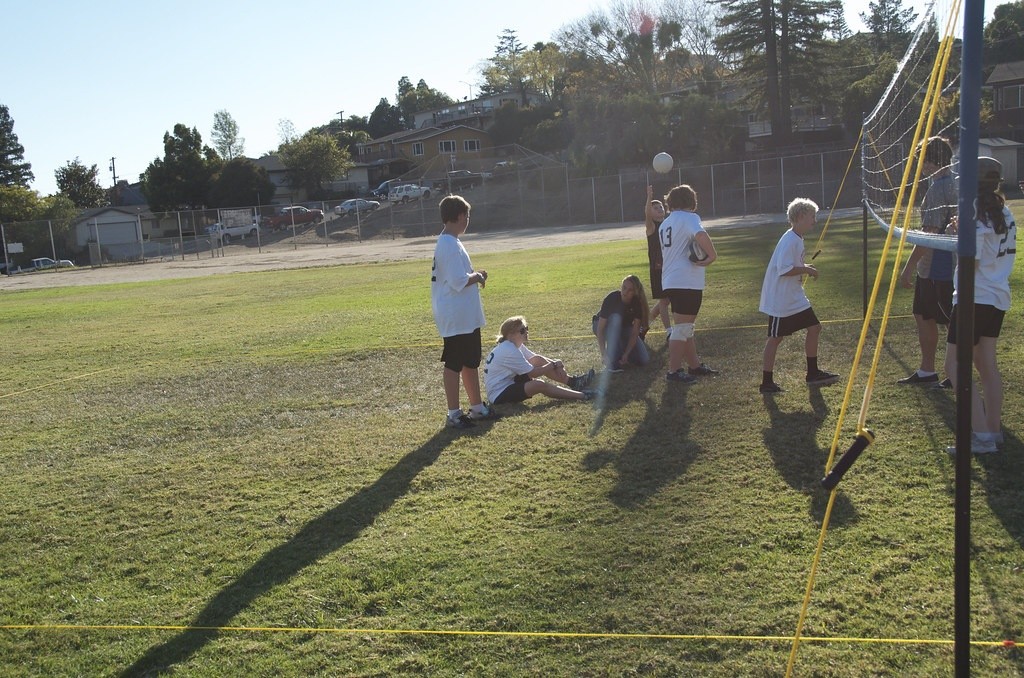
[954,157,1002,181]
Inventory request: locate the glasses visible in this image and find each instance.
[515,326,528,334]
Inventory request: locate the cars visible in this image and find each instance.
[12,257,74,275]
[439,170,484,190]
[273,206,324,231]
[387,184,430,205]
[209,218,261,243]
[334,198,380,217]
[373,177,404,200]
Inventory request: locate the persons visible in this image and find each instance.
[638,172,673,345]
[658,184,721,384]
[431,193,502,429]
[758,198,839,393]
[897,136,964,389]
[945,156,1016,455]
[484,315,596,406]
[592,274,651,372]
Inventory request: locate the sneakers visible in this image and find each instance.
[666,368,699,384]
[572,370,594,392]
[467,402,503,420]
[760,382,784,391]
[806,370,840,384]
[445,411,477,428]
[583,391,597,401]
[688,363,720,376]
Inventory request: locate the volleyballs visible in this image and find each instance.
[652,152,673,174]
[688,241,707,262]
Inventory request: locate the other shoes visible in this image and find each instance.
[896,372,939,383]
[638,326,648,342]
[931,379,953,391]
[991,432,1003,444]
[946,438,997,454]
[666,333,672,341]
[608,364,624,373]
